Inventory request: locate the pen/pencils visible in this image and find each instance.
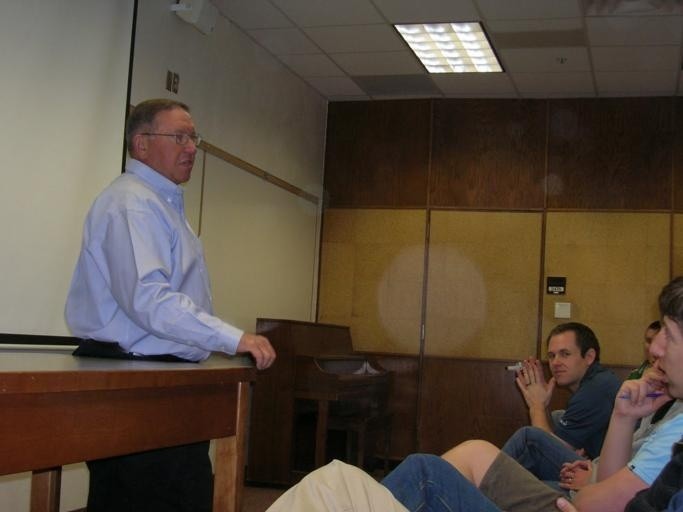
[505,366,523,371]
[618,394,665,399]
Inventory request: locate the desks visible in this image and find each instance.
[0,351,258,512]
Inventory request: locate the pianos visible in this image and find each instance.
[243,317,395,487]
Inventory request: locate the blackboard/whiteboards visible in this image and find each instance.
[126,103,320,358]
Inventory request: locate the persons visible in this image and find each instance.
[63,97,276,512]
[264,275,682,511]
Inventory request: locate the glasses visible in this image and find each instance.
[142,130,202,146]
